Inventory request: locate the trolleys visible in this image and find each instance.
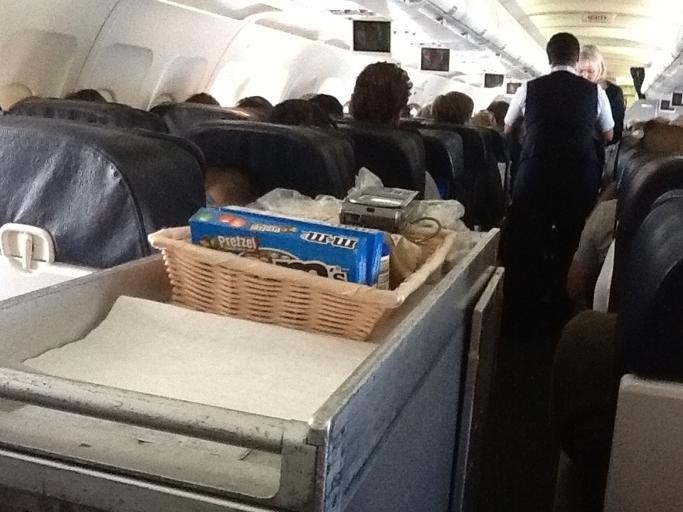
[0,226,505,511]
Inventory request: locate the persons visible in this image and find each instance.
[550,115,683,512]
[65,85,107,103]
[502,32,618,352]
[185,93,344,127]
[350,62,510,200]
[575,42,625,147]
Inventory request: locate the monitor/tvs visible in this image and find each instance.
[658,99,676,113]
[421,47,450,71]
[353,20,391,53]
[670,92,683,108]
[507,83,521,94]
[485,74,504,88]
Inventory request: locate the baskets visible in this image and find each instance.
[145,224,457,343]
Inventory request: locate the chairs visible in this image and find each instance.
[182,116,354,204]
[148,97,247,132]
[322,96,509,195]
[1,113,212,264]
[604,133,683,387]
[4,96,171,150]
